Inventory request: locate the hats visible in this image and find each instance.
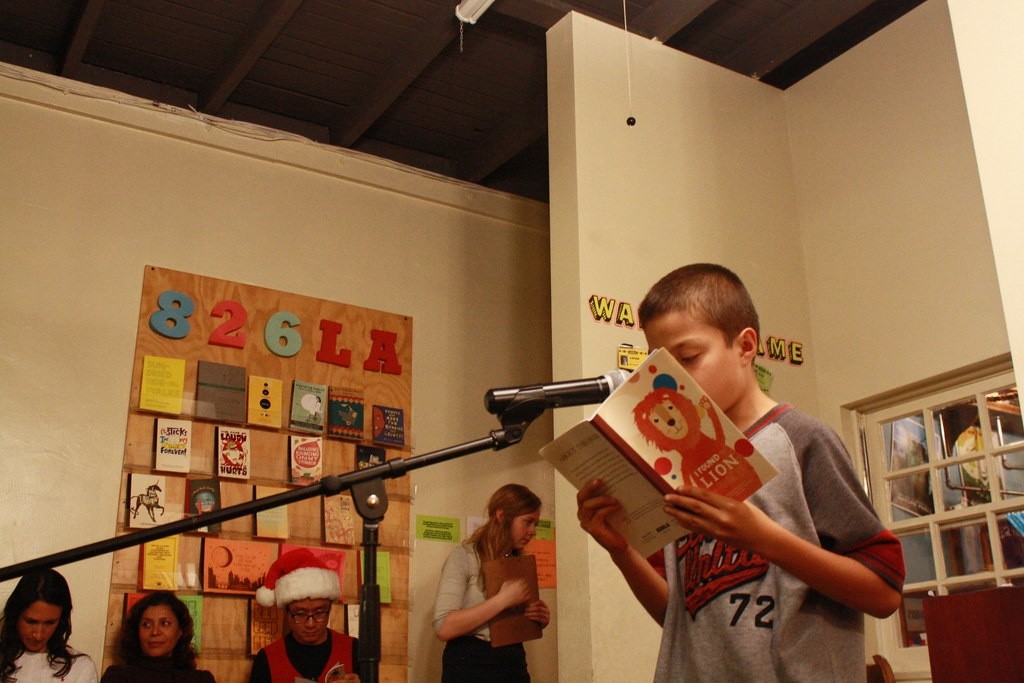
[256,547,344,609]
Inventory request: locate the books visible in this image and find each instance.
[122,593,359,657]
[483,555,543,648]
[140,356,404,484]
[295,662,345,683]
[125,474,392,603]
[539,347,777,558]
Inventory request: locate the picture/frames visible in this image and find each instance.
[956,426,1007,508]
[890,419,941,517]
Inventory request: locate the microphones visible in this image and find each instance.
[483,369,631,414]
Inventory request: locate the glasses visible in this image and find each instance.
[286,602,331,623]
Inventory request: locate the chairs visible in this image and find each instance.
[872,653,897,683]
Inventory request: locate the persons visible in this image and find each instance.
[432,484,550,683]
[577,263,905,682]
[0,569,98,683]
[99,591,215,683]
[247,548,361,683]
